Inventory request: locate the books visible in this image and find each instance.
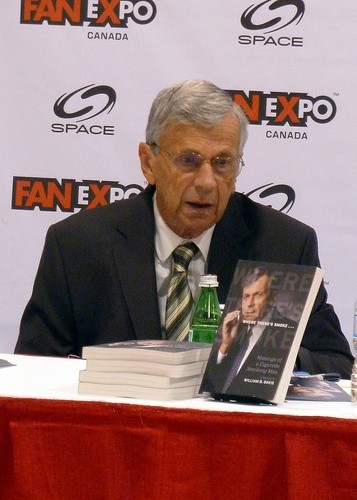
[198,260,325,405]
[78,339,213,400]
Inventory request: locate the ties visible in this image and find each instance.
[220,329,253,394]
[164,241,200,341]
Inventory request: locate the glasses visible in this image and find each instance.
[149,142,245,178]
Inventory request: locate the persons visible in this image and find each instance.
[198,265,298,399]
[288,377,343,400]
[13,79,355,380]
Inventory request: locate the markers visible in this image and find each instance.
[299,372,340,381]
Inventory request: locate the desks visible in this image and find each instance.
[0,354,357,500]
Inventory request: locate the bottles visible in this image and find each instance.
[349,297,357,408]
[187,274,222,344]
